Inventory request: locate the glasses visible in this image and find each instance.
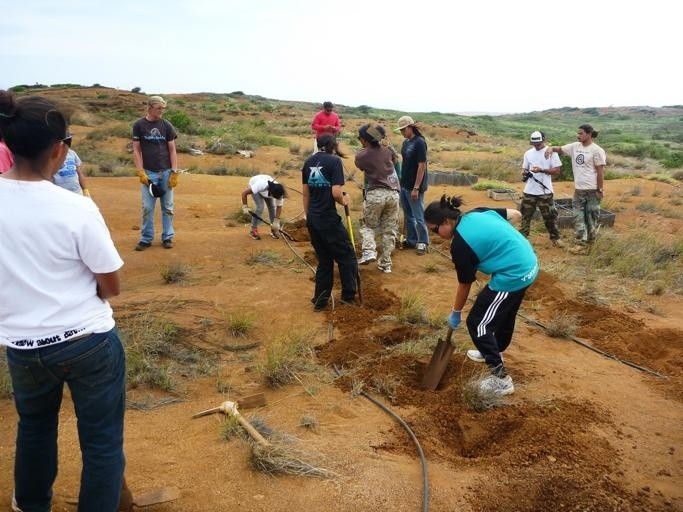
[432,224,439,234]
[56,135,73,147]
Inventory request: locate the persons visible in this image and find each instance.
[0,138,14,174]
[423,194,540,397]
[299,101,431,311]
[53,148,91,197]
[519,130,566,248]
[544,124,607,255]
[132,95,179,251]
[0,90,126,512]
[242,174,289,239]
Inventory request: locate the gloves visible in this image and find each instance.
[136,169,150,186]
[449,307,462,329]
[242,205,252,216]
[169,172,178,188]
[272,218,280,232]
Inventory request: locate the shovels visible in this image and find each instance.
[249,210,300,242]
[342,191,363,304]
[422,327,456,391]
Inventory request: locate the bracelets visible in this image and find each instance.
[542,168,544,173]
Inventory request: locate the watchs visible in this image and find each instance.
[597,188,603,193]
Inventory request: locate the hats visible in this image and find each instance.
[393,116,414,131]
[529,131,545,145]
[148,179,165,197]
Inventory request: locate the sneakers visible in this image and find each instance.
[136,241,151,251]
[568,240,596,256]
[249,231,260,240]
[357,249,377,265]
[162,239,173,248]
[378,265,392,273]
[481,375,515,398]
[552,239,565,248]
[416,243,428,256]
[467,350,504,362]
[269,229,279,239]
[396,239,407,250]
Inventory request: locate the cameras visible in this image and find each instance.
[521,168,533,182]
